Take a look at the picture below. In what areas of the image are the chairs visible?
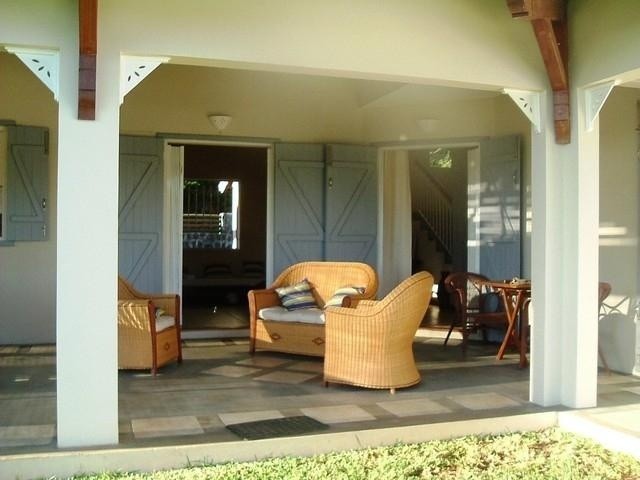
[118,277,182,380]
[442,271,508,355]
[323,270,434,390]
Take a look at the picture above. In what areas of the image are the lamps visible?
[209,115,231,130]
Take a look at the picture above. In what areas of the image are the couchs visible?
[248,260,378,361]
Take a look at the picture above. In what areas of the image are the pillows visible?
[201,261,233,278]
[274,277,364,314]
[243,258,264,278]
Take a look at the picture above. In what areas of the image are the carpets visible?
[226,414,326,439]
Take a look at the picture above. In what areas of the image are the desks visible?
[478,279,532,371]
